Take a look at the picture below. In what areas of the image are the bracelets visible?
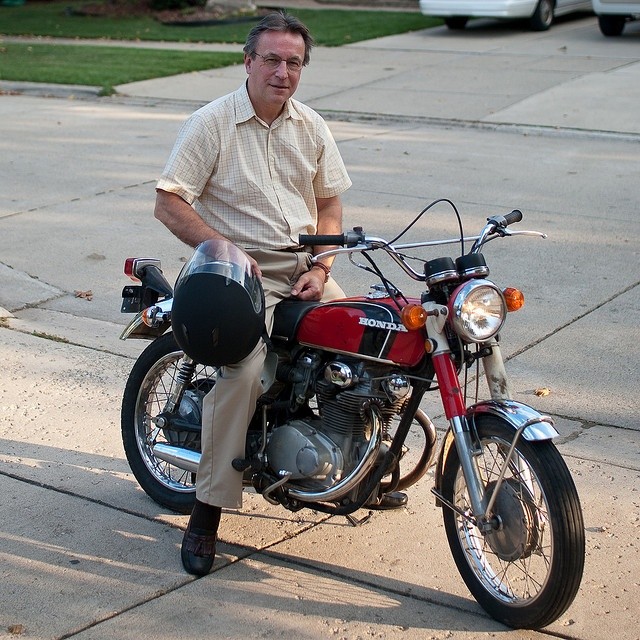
[313,262,329,284]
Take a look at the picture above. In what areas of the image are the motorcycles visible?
[119,199,585,629]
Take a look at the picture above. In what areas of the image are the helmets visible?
[171,239,265,366]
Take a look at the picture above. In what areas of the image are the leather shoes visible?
[181,500,221,575]
[382,491,407,506]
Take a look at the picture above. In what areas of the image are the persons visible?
[155,12,342,576]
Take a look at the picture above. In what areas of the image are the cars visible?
[419,0,595,31]
[593,0,640,35]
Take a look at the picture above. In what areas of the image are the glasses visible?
[251,52,306,73]
